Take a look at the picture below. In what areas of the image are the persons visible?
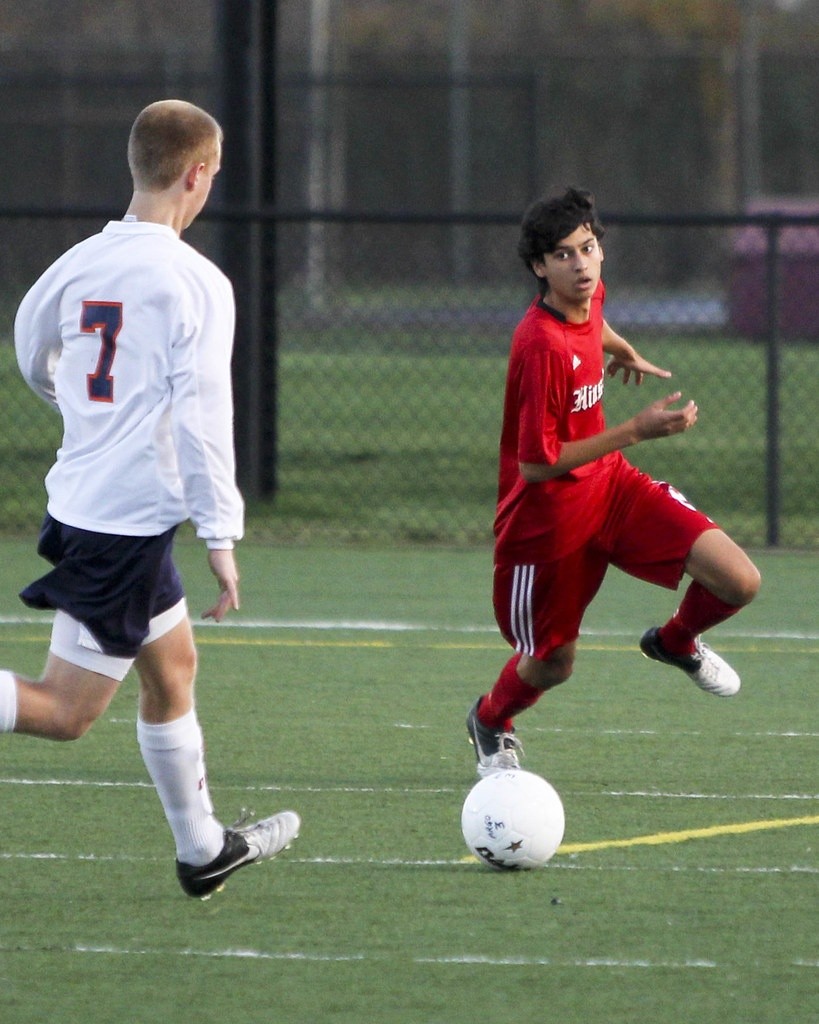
[466,188,763,779]
[0,98,302,899]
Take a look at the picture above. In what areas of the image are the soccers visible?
[461,770,565,869]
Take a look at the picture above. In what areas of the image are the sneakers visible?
[176,807,301,901]
[463,695,525,784]
[638,626,741,697]
